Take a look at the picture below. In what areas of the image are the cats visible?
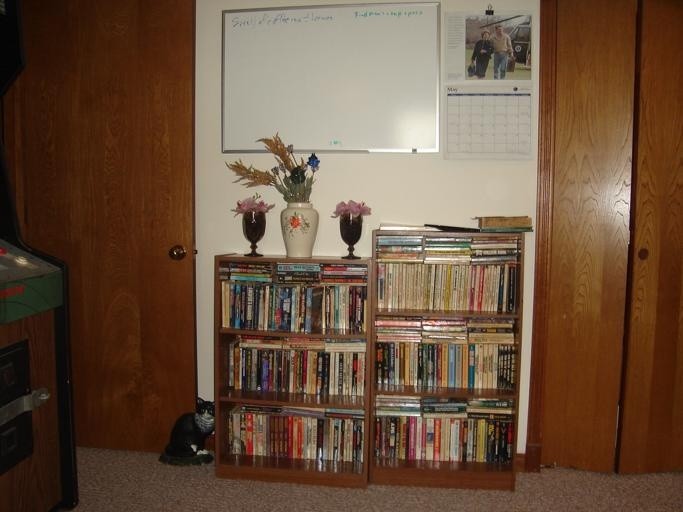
[158,396,215,466]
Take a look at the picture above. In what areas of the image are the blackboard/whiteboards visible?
[220,3,441,155]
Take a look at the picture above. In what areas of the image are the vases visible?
[280,203,319,258]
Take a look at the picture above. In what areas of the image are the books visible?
[373,394,517,465]
[223,403,365,464]
[374,317,518,391]
[223,334,367,407]
[376,215,533,263]
[376,261,520,314]
[219,261,369,334]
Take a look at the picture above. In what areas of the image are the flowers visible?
[222,132,372,218]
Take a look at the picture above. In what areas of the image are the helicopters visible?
[479,14,532,68]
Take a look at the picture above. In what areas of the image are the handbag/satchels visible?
[467,64,476,76]
[508,59,516,73]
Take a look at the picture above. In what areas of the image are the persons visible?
[489,25,515,80]
[467,30,492,78]
